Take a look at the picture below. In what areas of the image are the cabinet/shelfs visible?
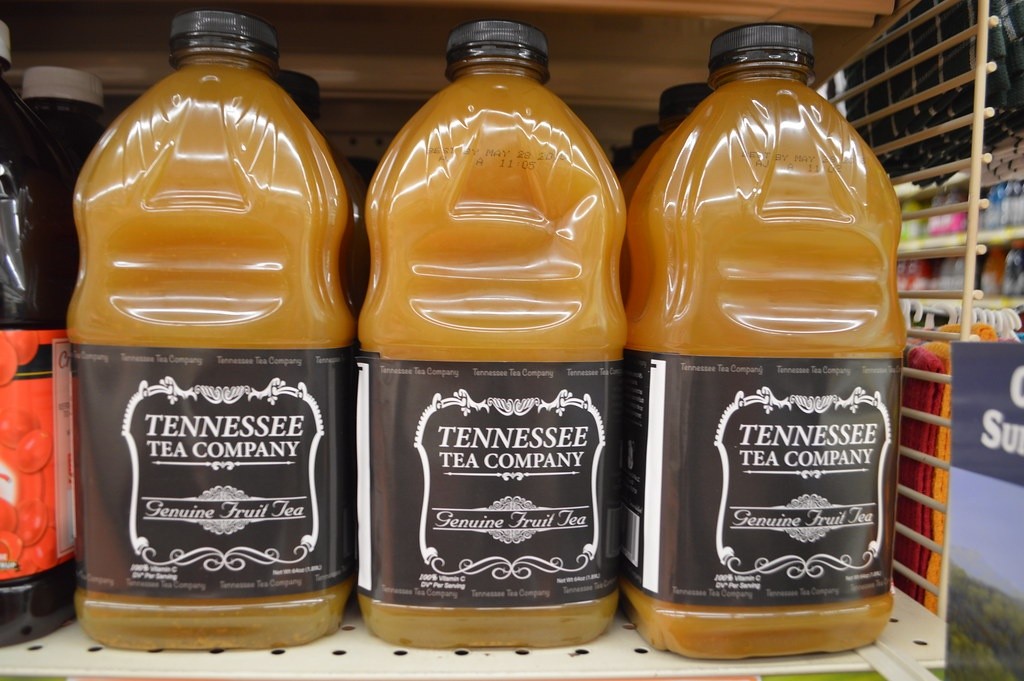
[893,160,1024,310]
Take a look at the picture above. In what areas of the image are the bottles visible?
[0,8,901,659]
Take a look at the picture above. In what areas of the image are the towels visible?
[837,1,1022,182]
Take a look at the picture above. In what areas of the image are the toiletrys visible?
[896,184,1024,294]
